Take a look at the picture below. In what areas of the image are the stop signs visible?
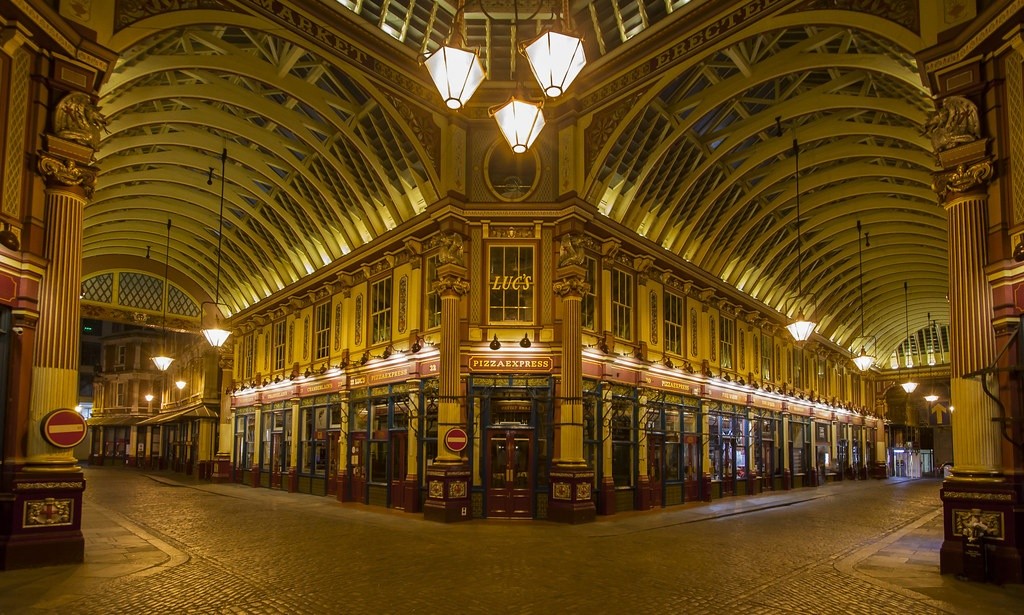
[445,427,468,452]
[43,407,88,449]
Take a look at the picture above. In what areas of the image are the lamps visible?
[490,333,502,350]
[599,130,957,420]
[144,145,429,399]
[417,0,590,156]
[517,331,532,349]
